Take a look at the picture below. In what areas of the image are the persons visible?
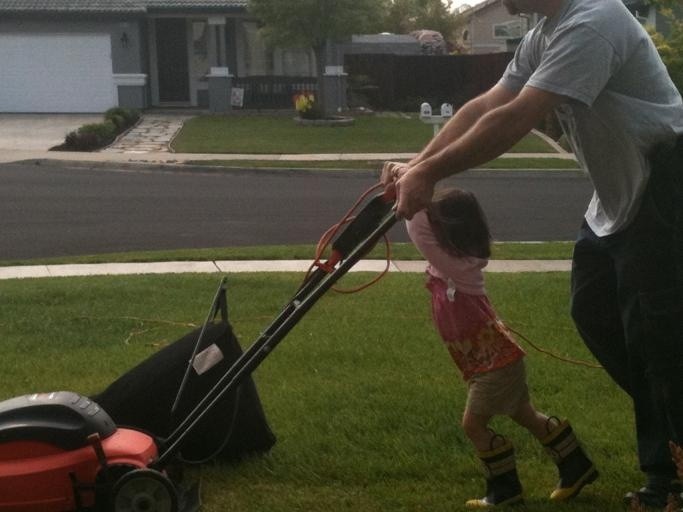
[391,165,598,508]
[382,0,683,512]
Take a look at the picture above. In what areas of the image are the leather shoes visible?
[624,486,682,509]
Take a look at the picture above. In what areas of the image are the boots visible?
[538,416,600,501]
[465,428,524,509]
